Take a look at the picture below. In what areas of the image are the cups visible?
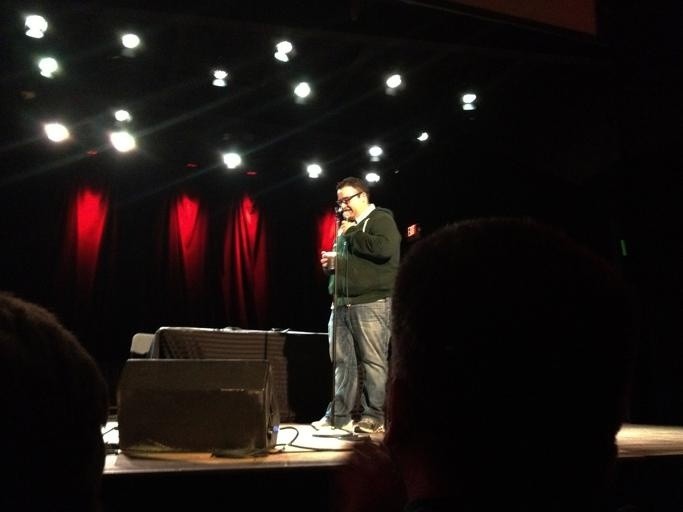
[324,251,338,270]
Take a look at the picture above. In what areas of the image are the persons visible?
[0,293,109,512]
[310,176,401,434]
[342,214,626,510]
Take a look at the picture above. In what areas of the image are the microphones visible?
[342,210,352,223]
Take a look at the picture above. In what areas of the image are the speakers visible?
[115,358,282,455]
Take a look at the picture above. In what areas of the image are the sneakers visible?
[311,416,354,431]
[354,416,385,434]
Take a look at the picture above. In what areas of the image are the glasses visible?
[335,192,362,205]
